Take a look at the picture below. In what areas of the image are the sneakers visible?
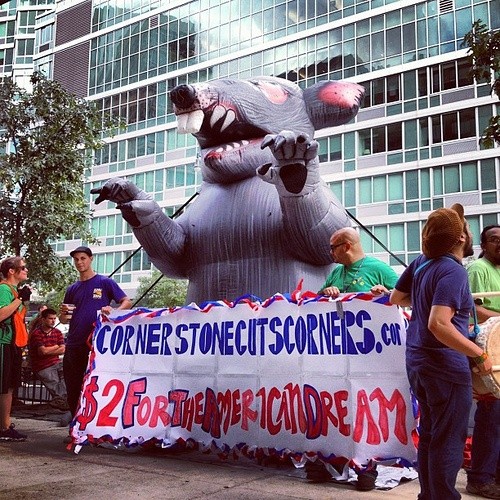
[0,428,28,441]
[306,463,346,483]
[356,469,379,490]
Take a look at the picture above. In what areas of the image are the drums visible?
[468,315,500,401]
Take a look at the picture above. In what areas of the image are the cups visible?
[61,304,74,320]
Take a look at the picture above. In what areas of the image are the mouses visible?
[90,75,365,309]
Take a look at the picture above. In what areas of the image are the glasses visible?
[330,243,347,251]
[14,263,27,271]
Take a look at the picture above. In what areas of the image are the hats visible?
[70,246,92,258]
[422,203,464,258]
